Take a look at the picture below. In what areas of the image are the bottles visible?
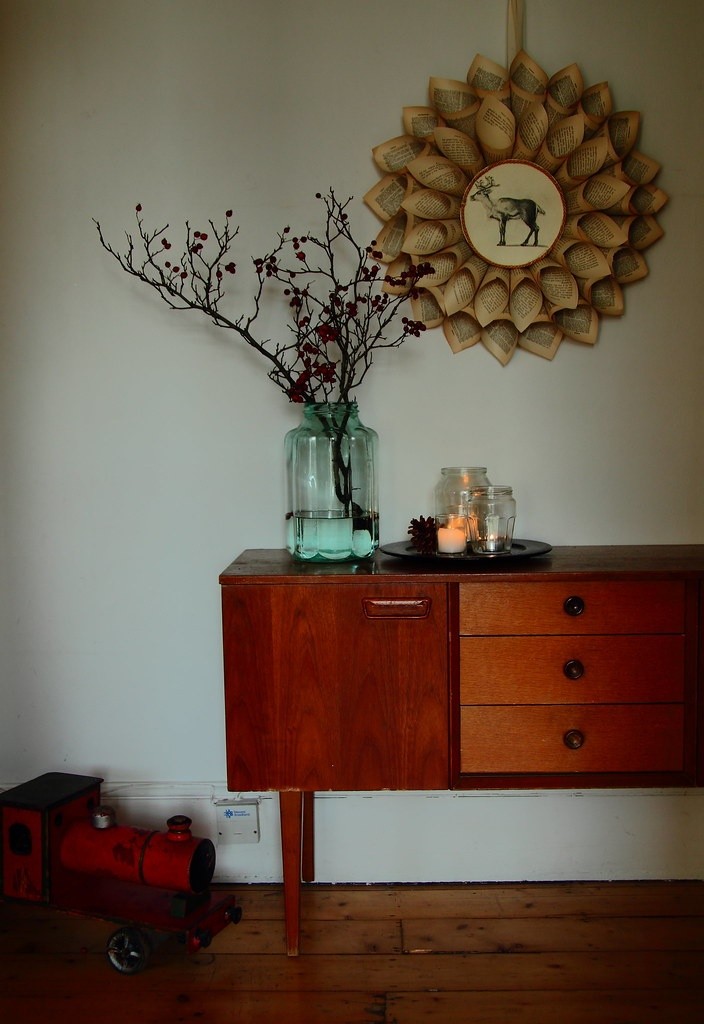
[467,486,517,555]
[434,465,493,545]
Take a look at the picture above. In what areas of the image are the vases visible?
[278,400,386,568]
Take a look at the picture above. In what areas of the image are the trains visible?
[0,772,242,974]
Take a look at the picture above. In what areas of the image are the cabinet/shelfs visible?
[216,543,703,960]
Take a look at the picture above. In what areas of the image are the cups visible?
[435,512,468,557]
